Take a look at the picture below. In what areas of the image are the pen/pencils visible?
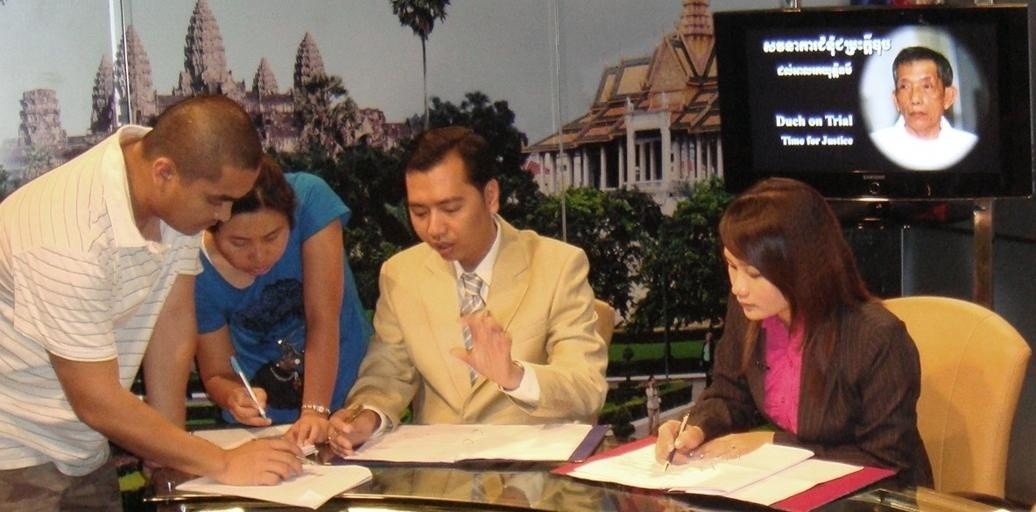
[229,355,268,421]
[328,402,364,441]
[664,409,692,472]
[295,456,320,467]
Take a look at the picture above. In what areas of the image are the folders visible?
[550,435,896,512]
[323,423,611,465]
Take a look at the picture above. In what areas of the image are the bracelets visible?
[298,402,331,415]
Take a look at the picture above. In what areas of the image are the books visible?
[174,458,375,512]
[345,419,594,462]
[191,428,326,460]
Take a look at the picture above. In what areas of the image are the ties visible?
[459,274,486,386]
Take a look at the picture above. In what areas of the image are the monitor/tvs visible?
[713,3,1036,202]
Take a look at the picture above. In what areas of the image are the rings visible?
[730,441,738,449]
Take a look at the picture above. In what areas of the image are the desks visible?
[0,425,1000,512]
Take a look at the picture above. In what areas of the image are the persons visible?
[652,174,938,492]
[324,122,611,463]
[0,89,312,512]
[190,153,371,452]
[865,39,983,175]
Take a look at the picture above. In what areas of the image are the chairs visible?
[867,293,1036,512]
